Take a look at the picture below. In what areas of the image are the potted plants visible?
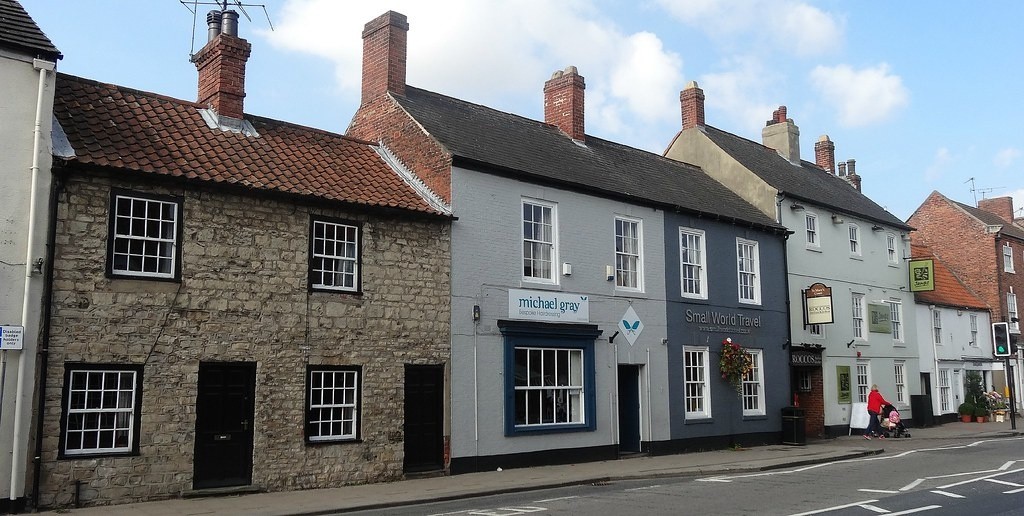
[975,408,985,423]
[982,410,990,423]
[958,402,974,423]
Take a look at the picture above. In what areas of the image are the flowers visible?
[713,338,754,397]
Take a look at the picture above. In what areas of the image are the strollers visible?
[877,404,912,438]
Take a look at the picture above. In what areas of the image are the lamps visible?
[788,201,811,211]
[803,343,810,348]
[832,213,844,225]
[995,237,1003,242]
[871,225,885,231]
[901,232,914,242]
[1003,317,1021,325]
[812,344,823,348]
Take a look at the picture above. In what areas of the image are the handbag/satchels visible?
[889,410,900,423]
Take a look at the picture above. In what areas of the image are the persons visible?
[981,391,1000,405]
[863,384,893,441]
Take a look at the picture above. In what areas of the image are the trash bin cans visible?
[781,406,807,446]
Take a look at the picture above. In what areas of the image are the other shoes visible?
[863,434,871,440]
[879,435,885,440]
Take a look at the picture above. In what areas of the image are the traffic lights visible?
[1009,333,1019,355]
[992,322,1012,357]
